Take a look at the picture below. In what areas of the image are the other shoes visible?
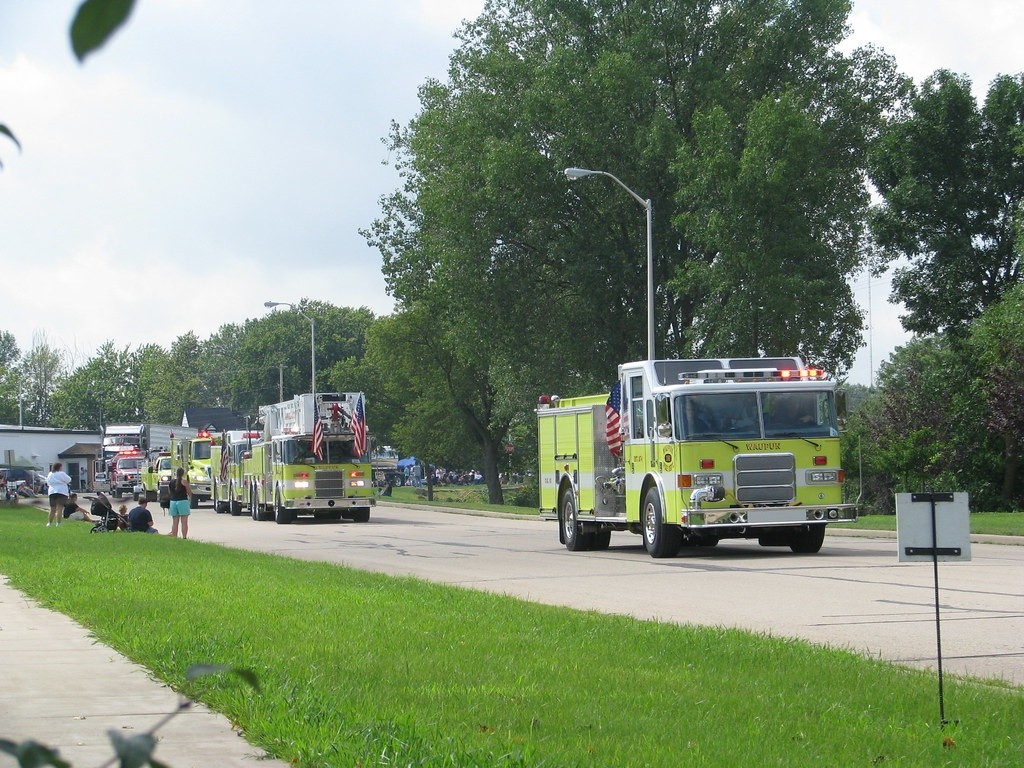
[46,524,51,527]
[56,525,60,527]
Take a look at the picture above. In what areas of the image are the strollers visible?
[83,491,132,533]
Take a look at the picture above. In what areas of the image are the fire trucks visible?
[244,388,371,524]
[533,356,863,558]
[94,393,263,516]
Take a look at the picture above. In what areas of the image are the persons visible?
[404,461,483,488]
[80,467,88,493]
[498,472,519,487]
[168,467,192,539]
[129,498,158,534]
[0,476,40,505]
[63,493,100,522]
[46,462,72,527]
[118,505,129,531]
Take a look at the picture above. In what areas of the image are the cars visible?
[376,468,406,487]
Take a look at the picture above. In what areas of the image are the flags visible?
[349,391,368,460]
[311,390,323,462]
[219,431,228,483]
[605,379,624,458]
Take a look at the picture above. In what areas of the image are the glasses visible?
[75,497,77,498]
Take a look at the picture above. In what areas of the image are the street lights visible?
[264,301,316,394]
[565,168,655,360]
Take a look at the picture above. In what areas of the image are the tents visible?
[397,456,435,479]
